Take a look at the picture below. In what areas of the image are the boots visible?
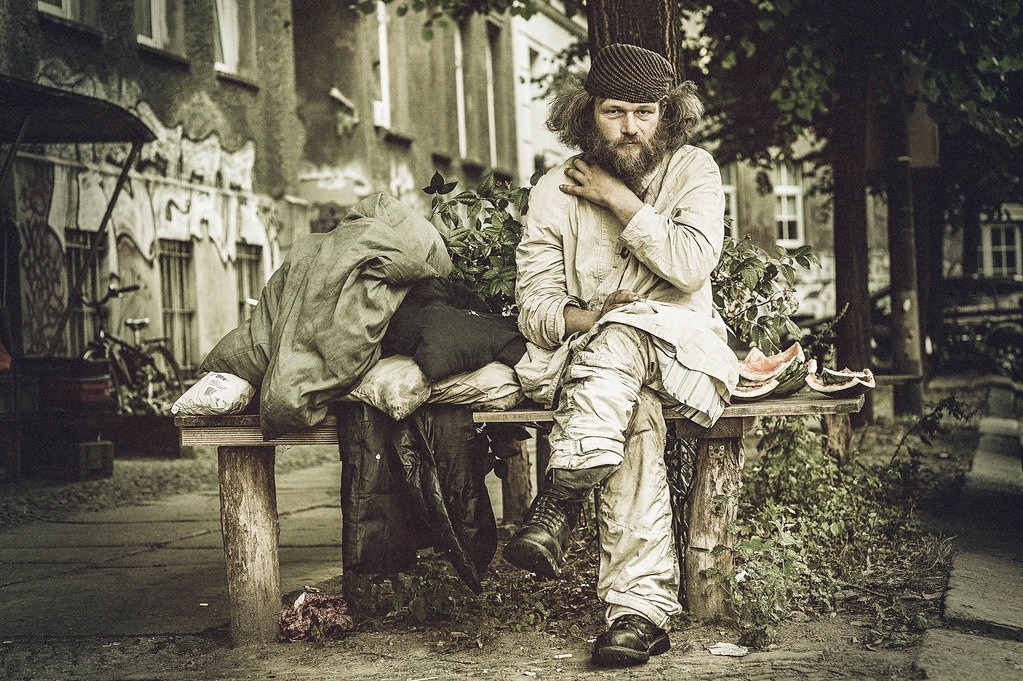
[501,468,588,579]
[591,614,671,666]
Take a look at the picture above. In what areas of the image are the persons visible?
[504,43,740,663]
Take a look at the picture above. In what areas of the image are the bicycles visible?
[76,282,187,418]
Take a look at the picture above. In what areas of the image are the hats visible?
[584,43,674,104]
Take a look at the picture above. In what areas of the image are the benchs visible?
[175,388,867,649]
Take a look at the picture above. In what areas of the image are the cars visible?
[775,270,1023,382]
